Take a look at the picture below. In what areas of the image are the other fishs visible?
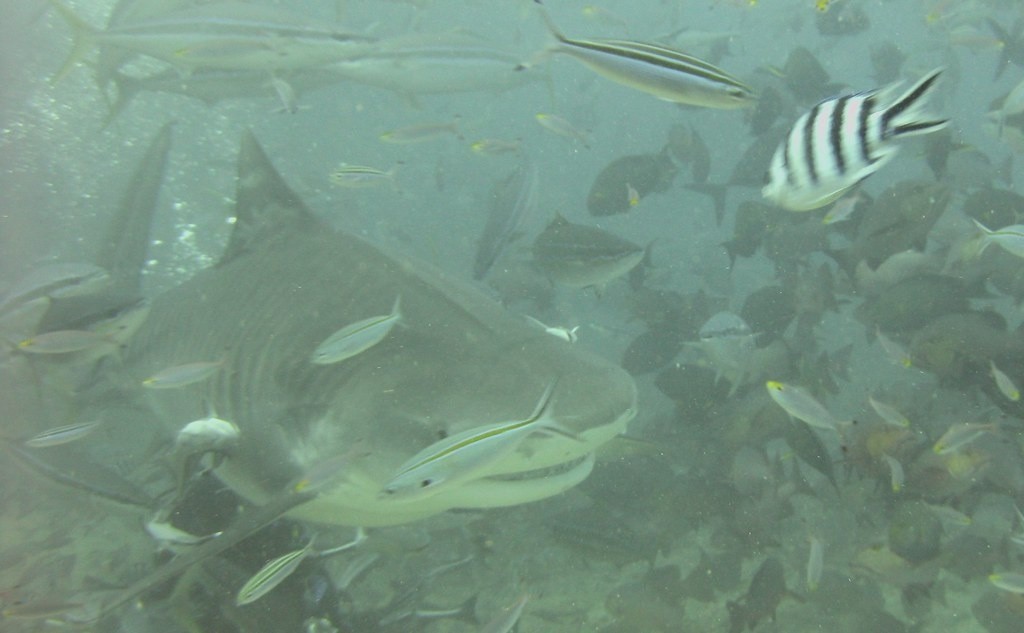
[0,0,1024,633]
